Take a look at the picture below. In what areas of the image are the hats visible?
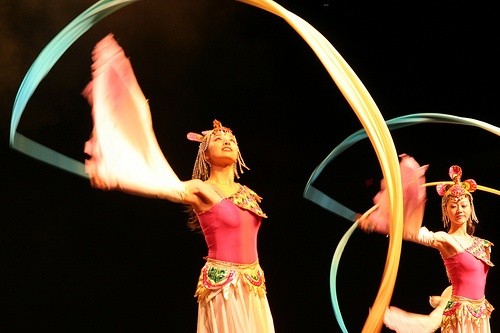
[436,164,479,223]
[186,118,250,179]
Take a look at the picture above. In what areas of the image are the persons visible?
[361,154,495,333]
[84,32,276,333]
